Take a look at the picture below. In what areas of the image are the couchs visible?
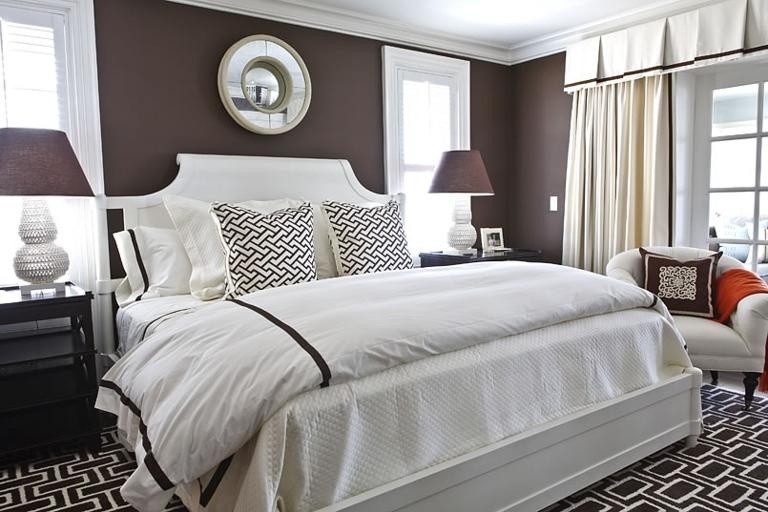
[605,244,768,409]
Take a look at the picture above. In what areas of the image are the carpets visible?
[2,379,768,511]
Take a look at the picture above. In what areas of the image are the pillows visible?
[321,200,409,277]
[208,199,317,302]
[162,192,335,301]
[115,225,189,307]
[637,246,724,315]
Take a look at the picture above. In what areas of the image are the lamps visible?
[428,149,496,255]
[0,127,95,299]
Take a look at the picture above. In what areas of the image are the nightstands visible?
[419,250,540,269]
[0,282,100,467]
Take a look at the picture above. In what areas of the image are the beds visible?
[95,155,703,511]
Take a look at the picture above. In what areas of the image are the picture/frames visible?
[478,227,504,252]
